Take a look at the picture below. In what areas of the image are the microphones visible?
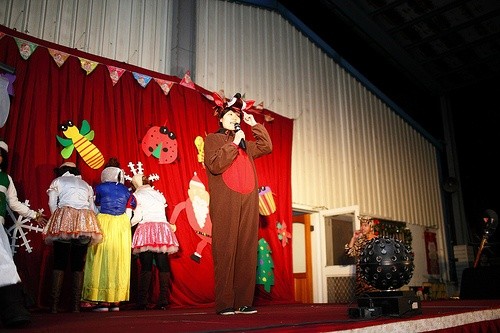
[234,123,246,149]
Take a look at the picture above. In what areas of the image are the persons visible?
[344,213,378,294]
[83,158,136,313]
[42,162,102,316]
[130,172,179,310]
[0,140,48,326]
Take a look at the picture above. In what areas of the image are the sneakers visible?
[234,306,257,314]
[220,309,235,315]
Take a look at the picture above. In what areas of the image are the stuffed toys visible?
[204,102,274,315]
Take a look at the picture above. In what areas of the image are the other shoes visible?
[110,306,120,311]
[0,284,31,326]
[93,308,109,311]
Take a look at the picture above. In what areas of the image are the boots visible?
[72,272,84,312]
[136,271,151,310]
[155,271,170,309]
[50,270,64,313]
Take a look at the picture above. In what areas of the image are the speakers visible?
[460,264,500,300]
